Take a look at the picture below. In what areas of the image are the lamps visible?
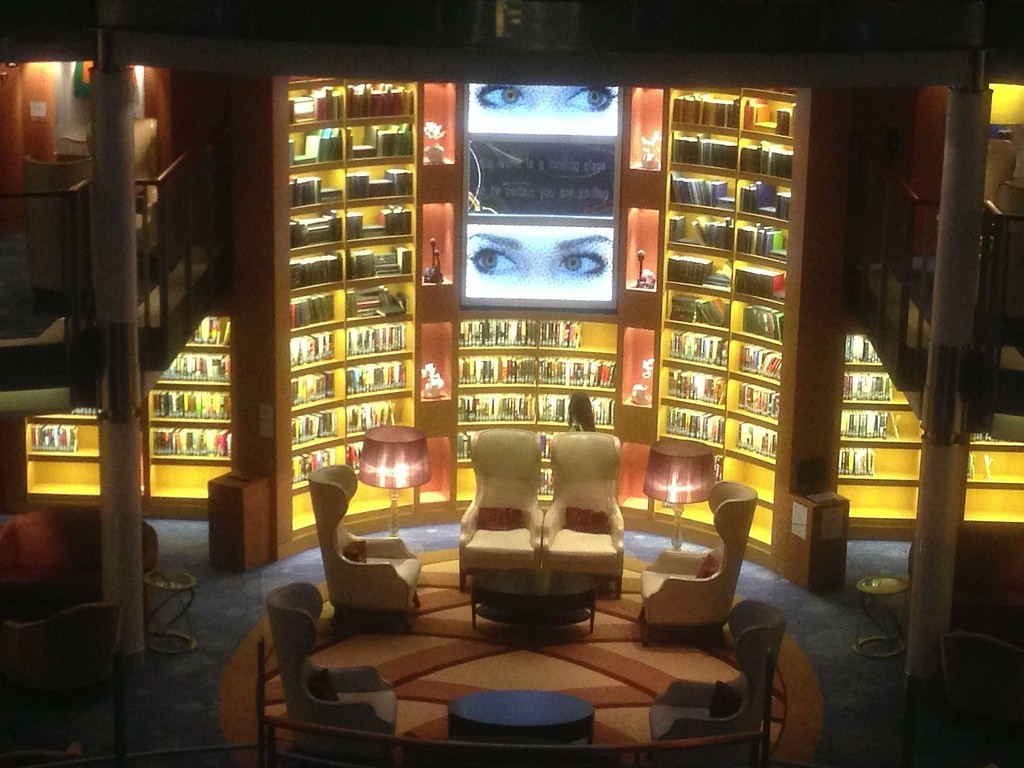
[357,426,430,537]
[643,439,716,554]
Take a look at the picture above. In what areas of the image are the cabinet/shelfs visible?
[230,74,850,565]
[838,334,1024,538]
[25,316,231,497]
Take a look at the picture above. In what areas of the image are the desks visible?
[852,575,908,658]
[469,566,598,633]
[446,688,595,744]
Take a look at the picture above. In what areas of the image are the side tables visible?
[142,567,199,653]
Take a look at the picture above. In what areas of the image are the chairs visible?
[457,428,625,599]
[905,524,1024,622]
[648,598,789,768]
[940,633,1024,740]
[267,582,397,764]
[639,481,758,649]
[1,602,121,760]
[308,465,423,635]
[0,502,158,616]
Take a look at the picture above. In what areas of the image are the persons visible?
[468,83,619,137]
[465,224,615,301]
[569,392,595,433]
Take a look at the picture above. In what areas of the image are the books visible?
[141,315,231,456]
[287,76,412,483]
[460,321,614,499]
[838,335,893,475]
[31,424,79,451]
[967,432,999,482]
[666,90,797,463]
